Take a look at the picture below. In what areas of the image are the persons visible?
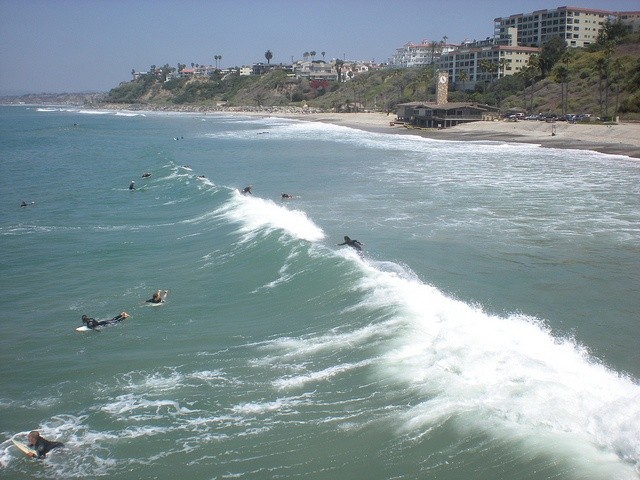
[24,431,65,460]
[21,201,28,206]
[140,172,151,177]
[241,184,254,196]
[80,312,129,329]
[335,235,364,253]
[280,192,294,200]
[128,180,135,190]
[146,289,169,304]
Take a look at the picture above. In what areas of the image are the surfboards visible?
[76,321,115,331]
[144,302,164,308]
[11,438,38,460]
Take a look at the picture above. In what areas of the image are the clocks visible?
[440,75,447,84]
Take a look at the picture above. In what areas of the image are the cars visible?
[504,112,601,122]
